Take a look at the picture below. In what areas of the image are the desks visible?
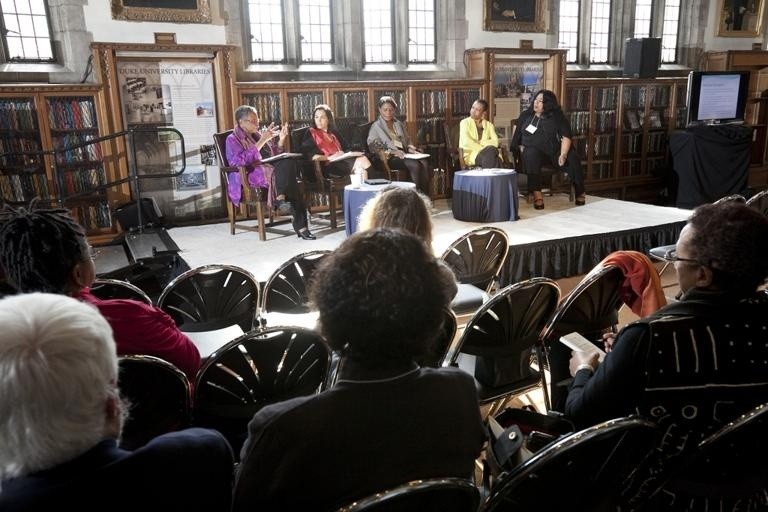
[668,123,755,209]
[452,167,521,223]
[343,180,416,235]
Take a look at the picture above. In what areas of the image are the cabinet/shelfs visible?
[232,78,491,220]
[0,82,133,247]
[565,77,692,193]
[704,50,768,172]
[464,48,570,192]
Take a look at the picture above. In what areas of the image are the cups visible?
[350,174,362,189]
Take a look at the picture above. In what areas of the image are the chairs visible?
[510,119,574,204]
[443,118,509,170]
[212,128,306,240]
[286,125,351,229]
[358,121,434,208]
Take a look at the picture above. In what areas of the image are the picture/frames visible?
[714,0,767,38]
[483,0,546,33]
[110,0,212,25]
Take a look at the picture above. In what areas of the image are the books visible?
[623,86,681,176]
[0,98,112,231]
[244,93,322,144]
[334,90,407,119]
[416,89,480,195]
[556,328,607,364]
[248,184,343,214]
[564,87,619,180]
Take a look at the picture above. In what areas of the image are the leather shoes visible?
[575,196,585,205]
[534,199,545,209]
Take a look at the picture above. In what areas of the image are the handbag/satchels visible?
[483,403,576,491]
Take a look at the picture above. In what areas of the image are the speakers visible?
[624,38,662,79]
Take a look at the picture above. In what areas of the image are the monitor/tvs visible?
[686,69,751,128]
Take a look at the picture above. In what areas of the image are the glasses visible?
[664,248,699,264]
[79,244,102,263]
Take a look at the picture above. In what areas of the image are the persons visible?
[356,185,435,249]
[222,103,319,241]
[459,99,504,168]
[0,291,235,511]
[299,103,380,186]
[367,95,430,197]
[0,192,201,378]
[567,201,767,444]
[510,90,586,210]
[234,227,487,512]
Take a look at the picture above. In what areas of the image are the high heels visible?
[296,229,316,240]
[272,199,292,214]
